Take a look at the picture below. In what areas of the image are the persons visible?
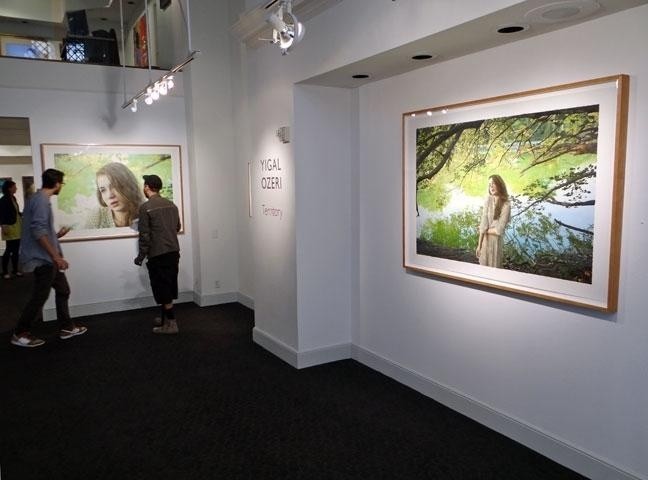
[476,175,510,268]
[134,174,181,334]
[9,169,88,348]
[0,181,23,281]
[85,162,146,230]
[134,27,142,66]
[24,180,35,198]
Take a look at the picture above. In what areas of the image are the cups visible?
[59,258,68,273]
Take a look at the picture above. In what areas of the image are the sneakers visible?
[11,332,46,347]
[153,319,179,334]
[60,326,87,340]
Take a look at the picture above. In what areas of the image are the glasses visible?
[59,182,66,187]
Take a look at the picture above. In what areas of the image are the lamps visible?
[265,0,305,57]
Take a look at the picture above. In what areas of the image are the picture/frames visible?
[400,72,629,317]
[39,142,185,244]
[130,6,151,67]
